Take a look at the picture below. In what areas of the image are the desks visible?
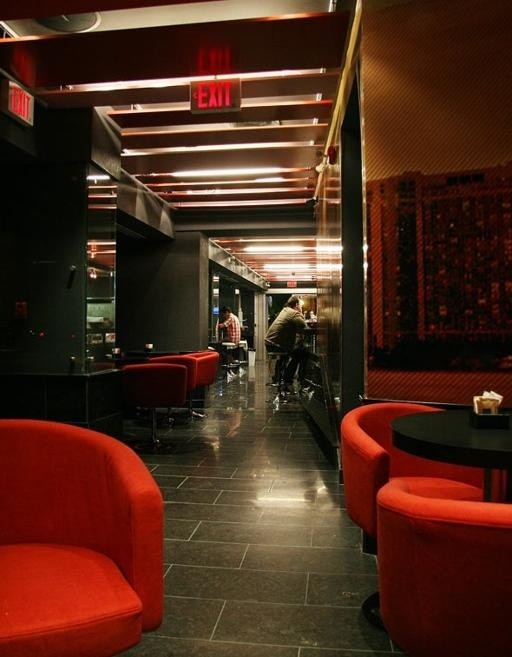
[391,407,510,503]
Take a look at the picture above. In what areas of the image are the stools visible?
[221,341,248,381]
[267,351,290,405]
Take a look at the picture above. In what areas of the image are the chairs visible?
[118,349,221,440]
[340,401,503,557]
[375,476,511,656]
[0,419,165,657]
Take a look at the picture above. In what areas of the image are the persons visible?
[218,305,241,363]
[265,295,307,383]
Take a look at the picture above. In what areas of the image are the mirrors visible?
[212,269,220,347]
[86,152,117,372]
